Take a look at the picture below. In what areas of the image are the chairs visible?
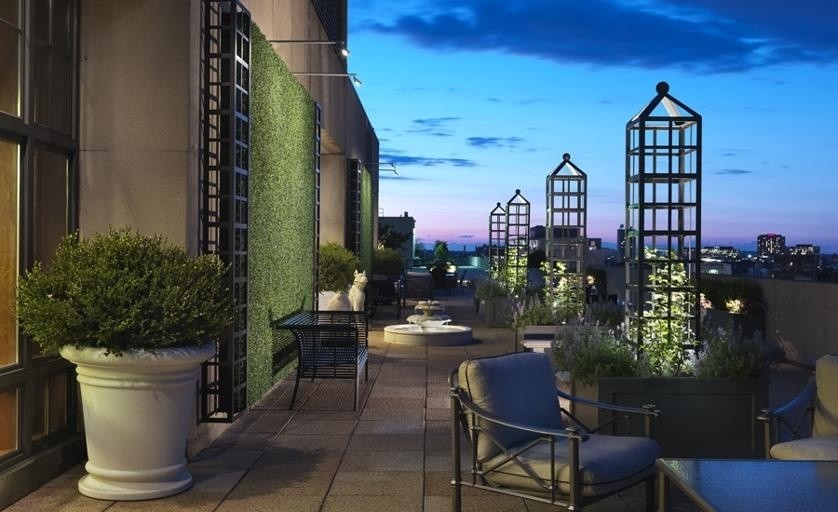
[280,304,370,412]
[441,349,664,512]
[368,260,468,320]
[756,352,838,461]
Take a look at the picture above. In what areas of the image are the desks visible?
[654,455,838,512]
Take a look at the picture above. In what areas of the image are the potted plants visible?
[314,241,357,310]
[17,225,238,498]
